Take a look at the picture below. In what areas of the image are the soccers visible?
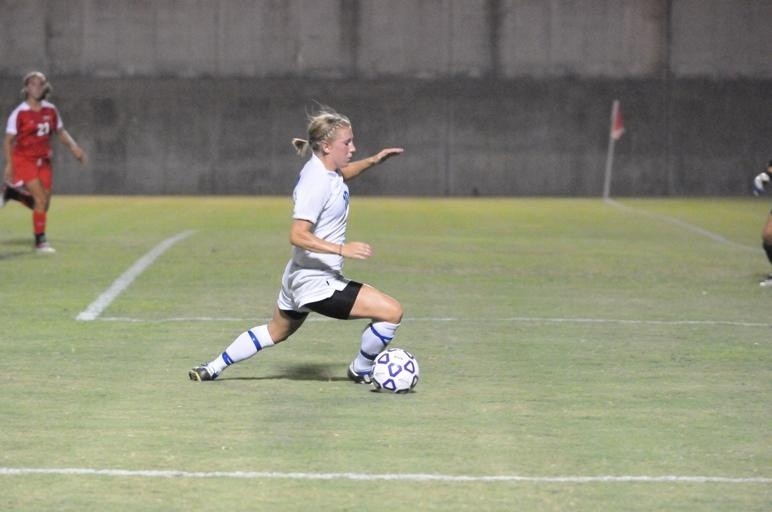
[370,347,419,394]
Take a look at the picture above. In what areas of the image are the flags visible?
[610,102,626,141]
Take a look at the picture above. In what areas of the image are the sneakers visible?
[760,273,772,288]
[189,366,215,382]
[346,363,373,385]
[0,182,10,208]
[33,243,57,254]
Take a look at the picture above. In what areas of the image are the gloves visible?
[751,173,771,197]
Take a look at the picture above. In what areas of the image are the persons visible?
[0,71,88,254]
[188,94,408,387]
[748,157,771,289]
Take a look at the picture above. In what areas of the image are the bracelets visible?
[339,244,343,256]
